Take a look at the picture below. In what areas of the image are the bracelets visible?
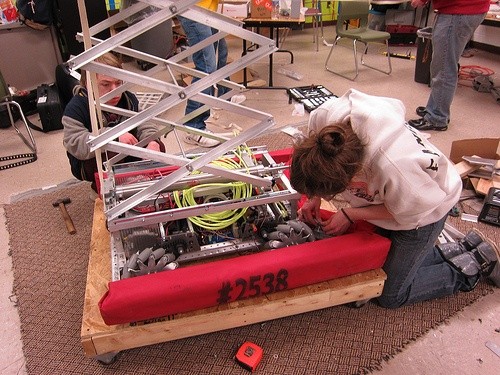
[342,208,354,224]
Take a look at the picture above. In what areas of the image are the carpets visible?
[4,125,500,375]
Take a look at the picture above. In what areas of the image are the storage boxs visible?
[251,0,279,47]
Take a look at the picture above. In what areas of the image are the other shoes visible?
[473,238,500,289]
[463,227,486,249]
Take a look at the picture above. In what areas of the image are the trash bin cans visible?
[121,0,175,70]
[415,26,432,84]
[368,10,385,31]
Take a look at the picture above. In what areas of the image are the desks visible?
[305,13,323,52]
[240,17,306,90]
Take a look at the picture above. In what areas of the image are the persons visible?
[177,0,246,147]
[62,53,166,203]
[407,0,492,132]
[291,87,500,309]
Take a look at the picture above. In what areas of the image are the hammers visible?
[52,197,76,234]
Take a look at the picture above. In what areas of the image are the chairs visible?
[0,71,37,154]
[325,0,391,81]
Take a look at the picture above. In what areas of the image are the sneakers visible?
[415,106,450,124]
[211,94,247,110]
[182,128,222,148]
[408,116,449,132]
